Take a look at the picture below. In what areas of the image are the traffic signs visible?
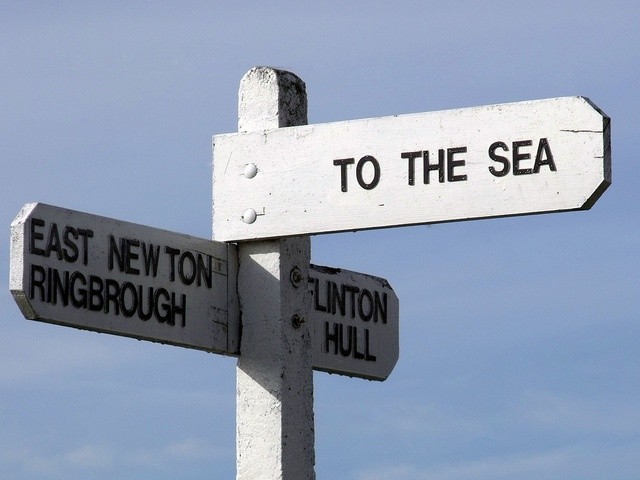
[212,96,612,243]
[9,201,398,381]
